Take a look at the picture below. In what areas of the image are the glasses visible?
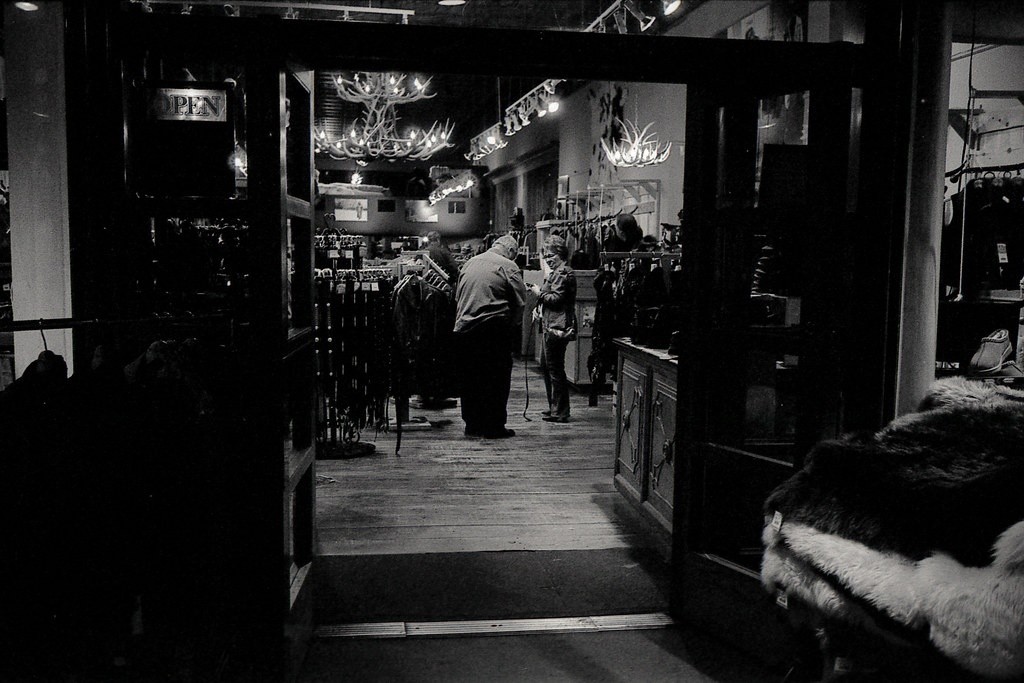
[543,252,558,260]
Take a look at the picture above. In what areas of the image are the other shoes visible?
[485,427,515,439]
[463,423,485,437]
[541,410,552,416]
[542,417,568,423]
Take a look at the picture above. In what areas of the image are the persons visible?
[531,236,577,424]
[612,213,649,253]
[453,236,527,440]
[423,231,456,283]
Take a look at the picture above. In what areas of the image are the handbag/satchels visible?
[544,291,578,343]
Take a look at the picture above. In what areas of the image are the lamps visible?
[661,0,681,16]
[542,80,561,94]
[463,133,508,160]
[510,112,522,131]
[530,98,547,118]
[538,93,559,113]
[599,83,672,168]
[314,0,456,161]
[625,0,655,32]
[504,117,516,137]
[518,107,530,126]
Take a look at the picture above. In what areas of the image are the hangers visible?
[394,264,430,294]
[38,318,54,360]
[423,259,451,300]
[144,311,202,377]
[603,250,683,272]
